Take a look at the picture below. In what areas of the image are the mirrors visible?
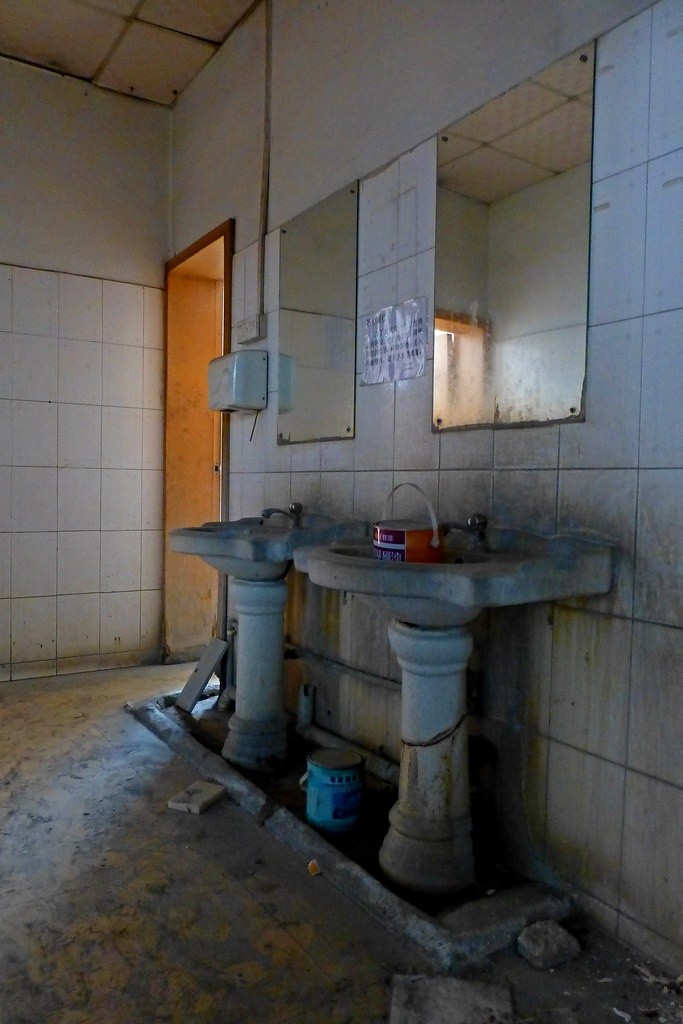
[431,39,596,432]
[276,179,360,446]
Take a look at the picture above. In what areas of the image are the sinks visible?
[166,517,366,581]
[290,537,614,623]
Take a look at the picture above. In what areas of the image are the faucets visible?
[458,513,486,548]
[260,502,301,527]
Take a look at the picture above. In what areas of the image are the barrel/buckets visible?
[372,482,444,564]
[299,748,364,834]
